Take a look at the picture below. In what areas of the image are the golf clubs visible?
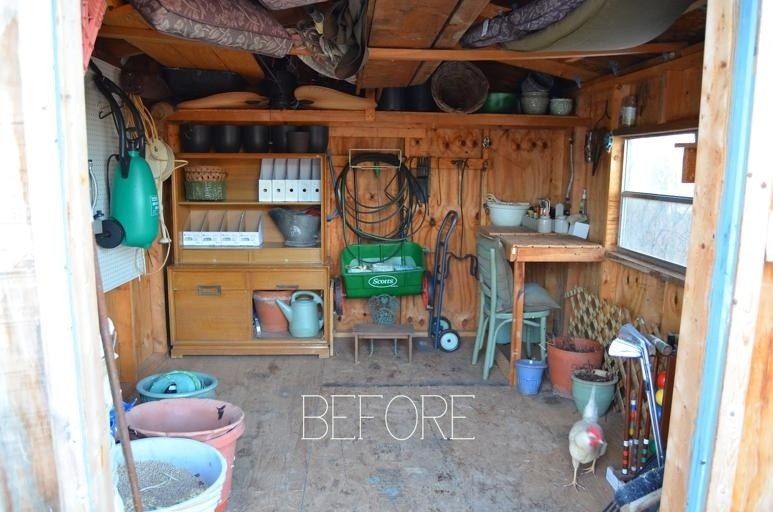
[608,322,663,468]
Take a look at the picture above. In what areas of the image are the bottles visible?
[621,96,636,128]
[528,197,550,219]
[563,192,570,215]
[536,214,552,234]
[578,188,587,216]
[554,216,568,233]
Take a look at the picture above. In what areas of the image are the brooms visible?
[612,467,665,512]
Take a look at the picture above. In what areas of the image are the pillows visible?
[503,281,562,312]
[475,233,514,305]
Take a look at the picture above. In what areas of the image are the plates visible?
[151,371,202,393]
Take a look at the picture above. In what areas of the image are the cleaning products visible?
[578,189,586,214]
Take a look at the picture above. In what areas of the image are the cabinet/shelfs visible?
[166,154,327,359]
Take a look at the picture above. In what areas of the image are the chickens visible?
[564,384,608,494]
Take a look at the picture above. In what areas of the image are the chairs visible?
[472,243,550,380]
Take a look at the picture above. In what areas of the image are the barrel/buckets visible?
[123,397,246,512]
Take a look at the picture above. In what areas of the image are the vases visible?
[253,290,294,332]
[180,123,329,154]
[431,59,573,115]
[547,337,603,394]
[111,370,247,512]
[571,369,618,417]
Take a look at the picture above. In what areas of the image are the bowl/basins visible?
[486,200,529,226]
[122,397,245,441]
[112,437,226,512]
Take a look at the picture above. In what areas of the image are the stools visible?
[352,324,415,362]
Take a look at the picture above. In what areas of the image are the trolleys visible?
[410,210,480,354]
[88,60,161,250]
[332,147,433,319]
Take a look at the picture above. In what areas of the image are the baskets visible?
[431,60,489,114]
[184,165,227,200]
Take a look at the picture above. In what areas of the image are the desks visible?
[475,225,607,386]
